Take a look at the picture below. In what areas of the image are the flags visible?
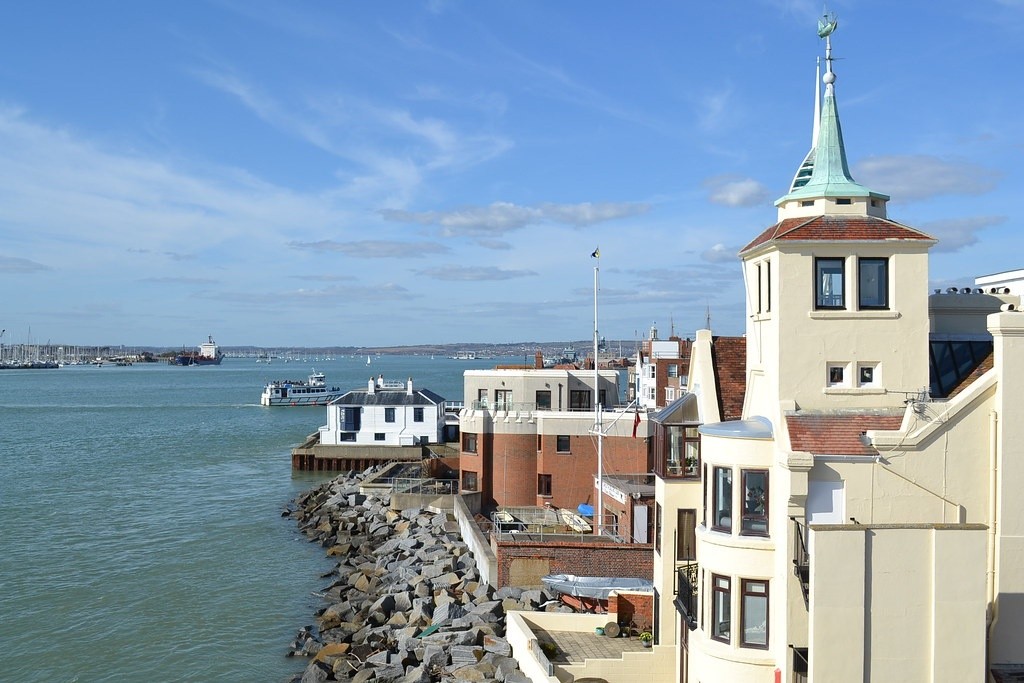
[591,249,599,258]
[632,412,641,439]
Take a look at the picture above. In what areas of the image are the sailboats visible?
[375,351,380,360]
[265,353,272,362]
[0,326,59,369]
[61,345,104,365]
[341,352,363,359]
[431,355,434,359]
[256,353,261,362]
[279,347,336,363]
[414,352,418,355]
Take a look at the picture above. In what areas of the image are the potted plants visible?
[745,487,762,513]
[680,457,693,472]
[638,631,652,646]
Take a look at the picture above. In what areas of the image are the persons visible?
[282,380,304,386]
[332,387,340,391]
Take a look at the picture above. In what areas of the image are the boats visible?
[541,574,653,600]
[366,355,371,367]
[117,359,132,366]
[490,511,514,523]
[175,349,198,365]
[561,508,592,533]
[195,335,225,365]
[261,370,344,406]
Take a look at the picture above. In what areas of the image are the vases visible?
[596,627,604,635]
